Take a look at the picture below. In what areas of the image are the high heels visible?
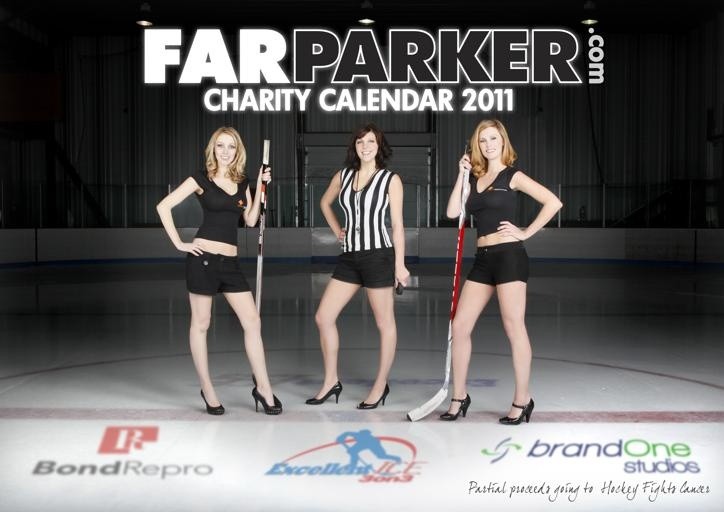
[305,380,343,405]
[251,386,282,416]
[356,383,390,410]
[497,397,535,426]
[439,393,471,421]
[200,388,225,416]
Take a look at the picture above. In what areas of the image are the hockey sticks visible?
[406,139,470,422]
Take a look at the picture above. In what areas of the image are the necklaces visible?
[355,168,380,233]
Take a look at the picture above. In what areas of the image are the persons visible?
[440,119,564,425]
[156,126,283,415]
[305,121,411,410]
